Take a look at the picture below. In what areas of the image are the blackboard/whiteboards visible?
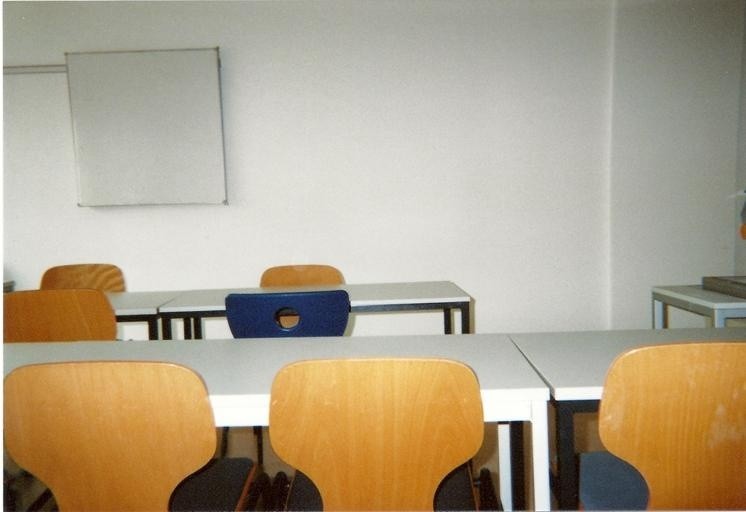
[64,46,227,207]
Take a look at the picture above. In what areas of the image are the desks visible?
[509,328,746,504]
[648,281,746,330]
[159,280,477,337]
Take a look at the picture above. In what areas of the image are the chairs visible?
[224,263,355,340]
[4,263,127,340]
[3,339,746,509]
